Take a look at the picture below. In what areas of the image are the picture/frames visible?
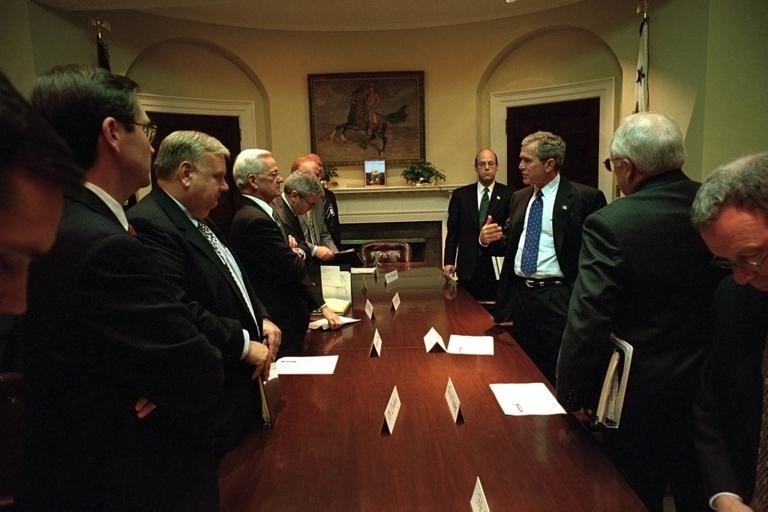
[305,69,427,169]
[363,160,388,187]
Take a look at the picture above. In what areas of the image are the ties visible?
[198,221,228,267]
[272,210,288,245]
[479,188,489,230]
[521,189,544,277]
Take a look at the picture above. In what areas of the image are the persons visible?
[362,78,379,140]
[442,148,519,301]
[267,168,327,291]
[0,70,84,319]
[26,64,233,510]
[227,148,343,343]
[292,157,341,264]
[126,129,286,467]
[304,153,342,255]
[687,149,767,511]
[477,130,608,394]
[552,113,717,510]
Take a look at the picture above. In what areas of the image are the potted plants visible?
[399,158,447,186]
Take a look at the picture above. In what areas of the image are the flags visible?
[632,18,649,113]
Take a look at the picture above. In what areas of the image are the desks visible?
[215,256,650,511]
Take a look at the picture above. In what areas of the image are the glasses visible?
[710,254,768,271]
[131,122,158,143]
[602,158,623,171]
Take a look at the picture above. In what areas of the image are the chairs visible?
[358,239,413,272]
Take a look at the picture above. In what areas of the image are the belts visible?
[519,279,562,288]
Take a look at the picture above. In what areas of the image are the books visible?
[309,262,354,319]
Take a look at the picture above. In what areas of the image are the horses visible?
[326,87,410,157]
